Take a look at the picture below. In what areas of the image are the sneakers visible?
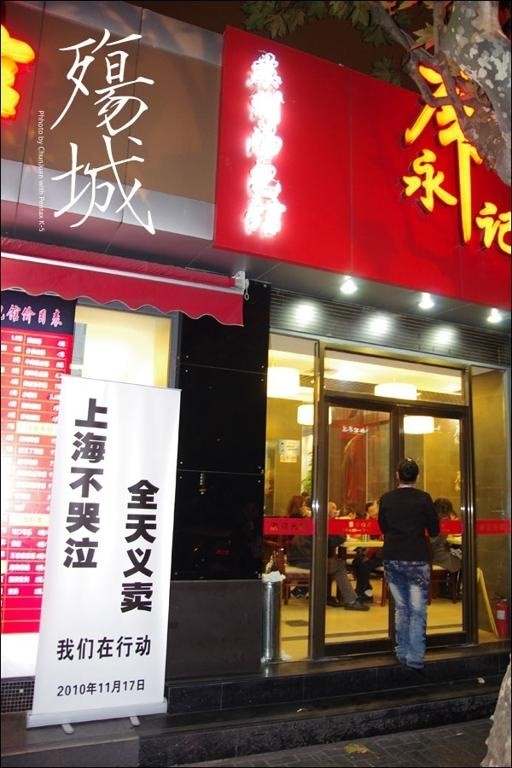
[397,656,424,669]
[288,585,374,611]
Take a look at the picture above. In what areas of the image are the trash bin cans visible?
[260,580,281,662]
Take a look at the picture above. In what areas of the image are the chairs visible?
[427,535,457,605]
[276,535,310,605]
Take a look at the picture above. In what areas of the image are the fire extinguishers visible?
[490,593,509,639]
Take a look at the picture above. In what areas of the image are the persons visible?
[281,489,464,610]
[378,456,442,675]
